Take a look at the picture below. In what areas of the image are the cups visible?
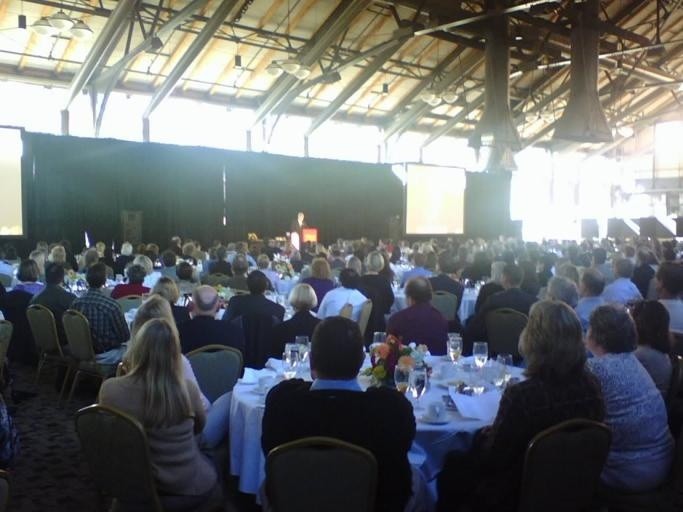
[372,332,386,344]
[282,352,298,379]
[440,363,459,380]
[429,401,445,419]
[258,376,273,391]
[284,343,298,362]
[410,367,427,412]
[473,342,487,370]
[446,333,460,361]
[450,337,462,366]
[394,365,410,396]
[497,354,512,379]
[296,336,309,362]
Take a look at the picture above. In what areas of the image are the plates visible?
[251,389,267,396]
[420,415,451,425]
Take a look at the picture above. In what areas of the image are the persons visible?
[249,254,279,291]
[213,240,226,259]
[111,265,150,299]
[387,275,449,356]
[98,318,228,497]
[160,250,176,278]
[623,246,638,265]
[557,263,578,284]
[182,243,197,266]
[301,242,313,263]
[95,240,105,257]
[145,250,160,270]
[222,255,248,291]
[127,294,212,415]
[360,237,368,256]
[427,250,463,322]
[167,236,182,256]
[376,239,393,258]
[148,277,189,323]
[535,256,554,277]
[590,248,611,276]
[78,249,99,274]
[348,256,362,277]
[288,211,309,237]
[147,243,158,253]
[202,248,218,271]
[287,257,302,297]
[662,241,677,261]
[0,244,19,287]
[208,247,230,275]
[116,242,135,264]
[631,250,655,299]
[133,254,161,288]
[481,298,604,512]
[177,285,247,379]
[50,246,66,263]
[361,251,394,352]
[626,298,674,399]
[172,262,192,293]
[408,244,425,266]
[653,261,683,332]
[400,254,432,287]
[228,242,255,267]
[345,241,366,267]
[13,258,45,296]
[538,275,578,311]
[318,268,368,322]
[584,302,675,495]
[67,264,126,365]
[271,284,321,358]
[302,259,337,311]
[30,263,78,343]
[602,257,643,309]
[221,269,285,338]
[574,269,606,329]
[474,261,506,315]
[479,265,535,322]
[261,239,282,258]
[29,250,46,274]
[260,316,421,512]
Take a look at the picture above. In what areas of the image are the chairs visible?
[0,320,13,385]
[185,344,244,402]
[431,290,457,321]
[72,403,162,512]
[61,308,95,405]
[26,303,71,397]
[265,436,379,511]
[359,300,373,338]
[519,418,612,511]
